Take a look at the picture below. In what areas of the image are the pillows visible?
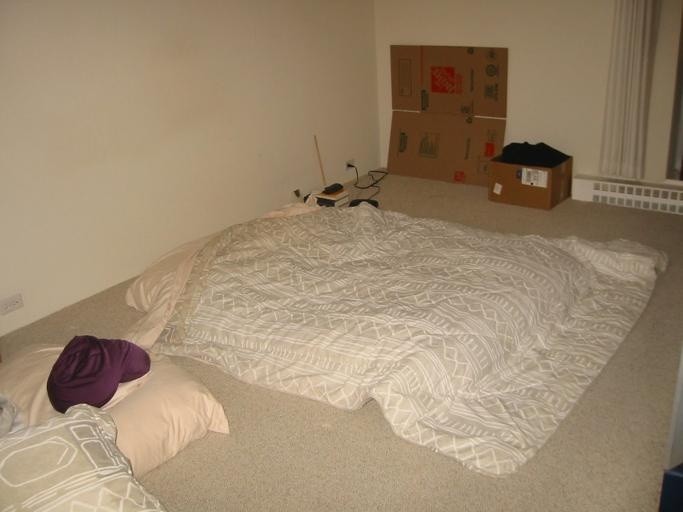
[12,340,230,482]
[1,400,162,510]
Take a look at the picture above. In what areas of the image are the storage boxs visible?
[487,144,573,211]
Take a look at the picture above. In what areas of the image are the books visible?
[316,189,349,207]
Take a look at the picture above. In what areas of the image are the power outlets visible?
[345,158,355,171]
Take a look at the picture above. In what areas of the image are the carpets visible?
[2,169,683,512]
